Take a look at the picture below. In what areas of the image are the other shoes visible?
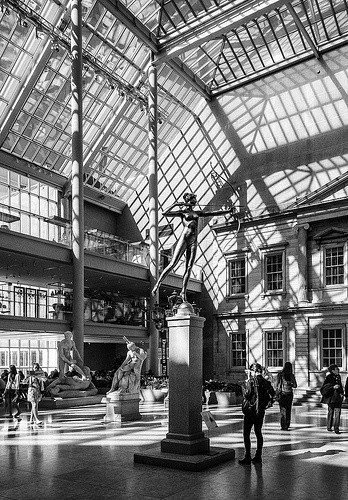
[28,421,34,425]
[37,421,42,425]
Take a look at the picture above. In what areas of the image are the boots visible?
[238,453,251,466]
[251,450,262,463]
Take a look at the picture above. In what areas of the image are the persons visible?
[276,362,297,431]
[238,364,276,464]
[46,331,96,393]
[151,193,236,303]
[320,365,344,434]
[23,363,47,425]
[0,369,154,391]
[106,342,147,395]
[4,364,22,418]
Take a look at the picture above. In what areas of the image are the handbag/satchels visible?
[274,388,282,403]
[242,397,258,416]
